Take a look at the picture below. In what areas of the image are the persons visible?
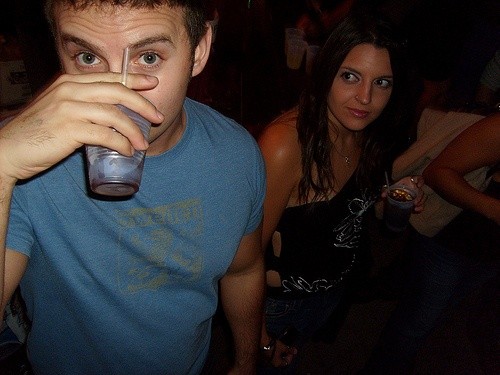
[257,20,432,375]
[357,111,500,375]
[0,0,267,375]
[471,50,500,111]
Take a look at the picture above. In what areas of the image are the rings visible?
[282,357,289,369]
[285,346,290,354]
[405,175,419,186]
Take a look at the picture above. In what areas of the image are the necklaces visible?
[327,135,362,166]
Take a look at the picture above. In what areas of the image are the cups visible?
[306,45,320,76]
[285,28,304,56]
[385,184,419,232]
[286,39,307,70]
[85,104,151,196]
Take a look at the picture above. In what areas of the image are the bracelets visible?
[259,335,277,363]
